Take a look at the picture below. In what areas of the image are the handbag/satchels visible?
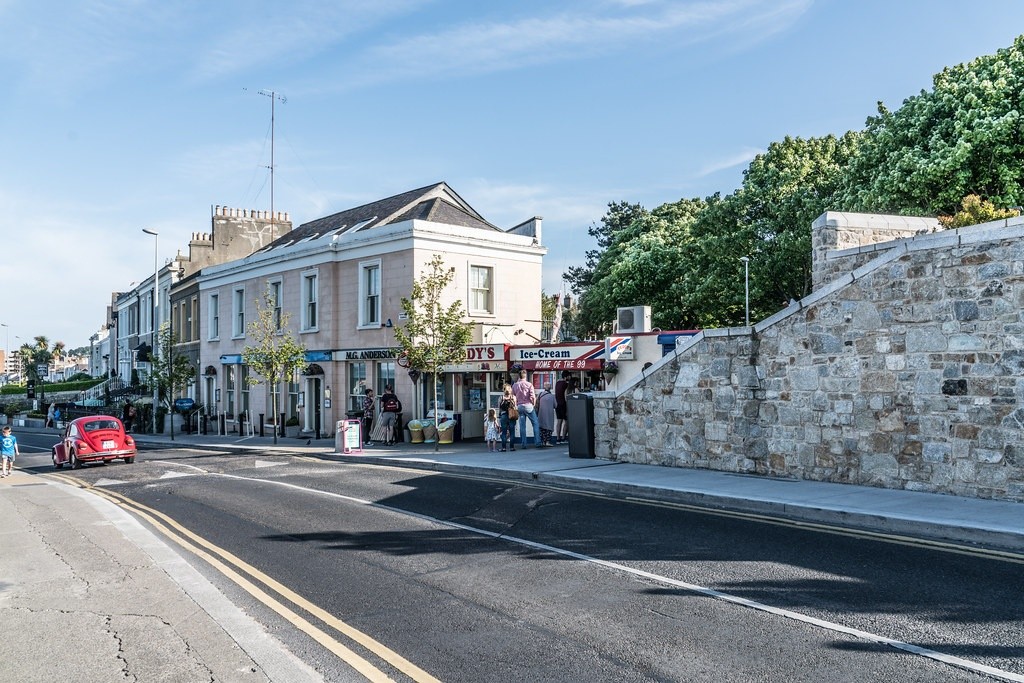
[508,396,518,420]
[535,392,542,417]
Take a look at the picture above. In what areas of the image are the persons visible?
[122,399,135,433]
[0,426,20,478]
[484,369,584,452]
[45,399,56,427]
[360,388,375,447]
[66,399,76,412]
[381,384,402,445]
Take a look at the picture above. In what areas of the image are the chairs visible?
[407,419,424,443]
[437,419,456,443]
[421,420,436,442]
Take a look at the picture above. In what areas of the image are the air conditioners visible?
[613,320,617,334]
[617,305,651,333]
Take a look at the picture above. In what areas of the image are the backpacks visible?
[129,406,136,416]
[385,394,397,411]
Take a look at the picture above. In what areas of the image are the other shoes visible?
[556,440,561,444]
[501,448,506,452]
[8,471,11,476]
[1,474,5,477]
[546,443,554,447]
[365,441,374,446]
[560,440,569,443]
[382,440,395,446]
[510,448,516,451]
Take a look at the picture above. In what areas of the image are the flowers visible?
[510,364,523,373]
[602,362,618,373]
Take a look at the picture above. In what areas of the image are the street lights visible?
[740,257,749,326]
[16,336,21,387]
[1,324,9,376]
[143,229,158,436]
[81,389,84,409]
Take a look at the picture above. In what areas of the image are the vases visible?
[510,373,520,383]
[603,372,615,385]
[409,371,421,385]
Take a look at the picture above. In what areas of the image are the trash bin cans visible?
[566,393,595,459]
[345,409,367,442]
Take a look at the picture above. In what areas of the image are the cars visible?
[52,416,137,470]
